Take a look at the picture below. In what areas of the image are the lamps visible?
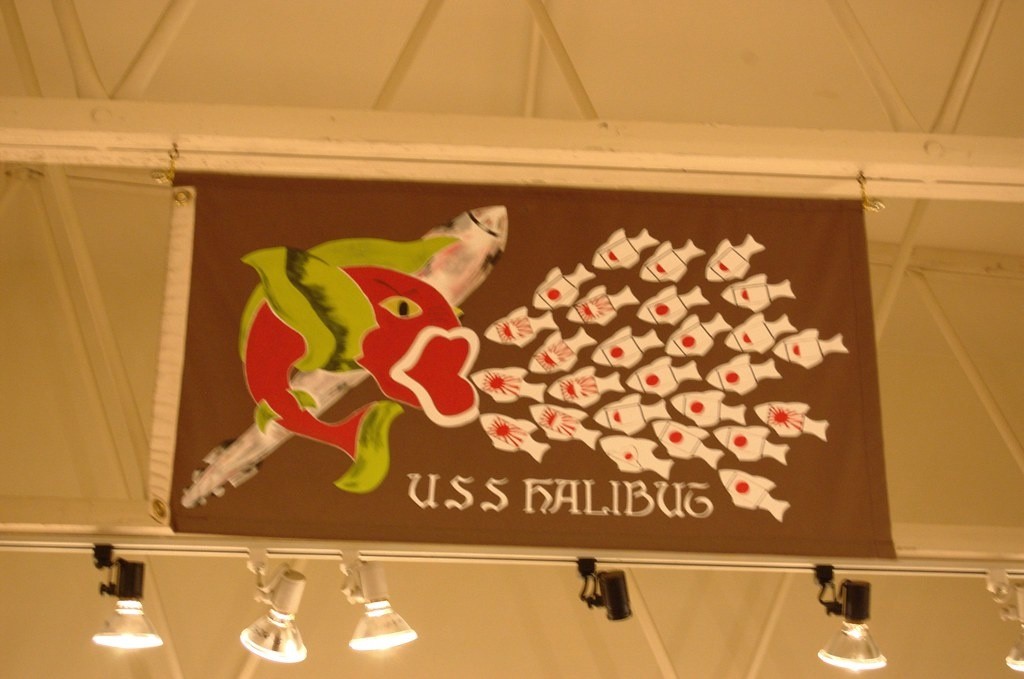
[239,543,306,662]
[986,568,1024,671]
[91,541,162,649]
[813,565,888,670]
[341,558,418,651]
[577,556,632,620]
